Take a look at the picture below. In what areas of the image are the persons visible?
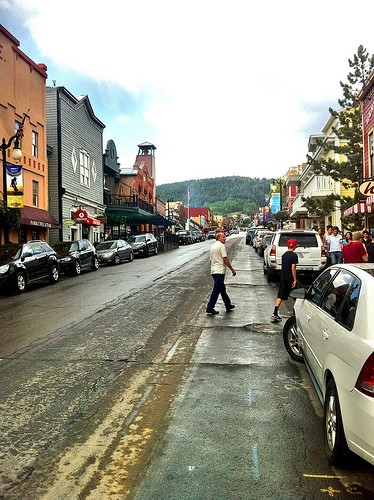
[324,289,337,309]
[313,225,374,269]
[271,240,301,321]
[206,233,235,316]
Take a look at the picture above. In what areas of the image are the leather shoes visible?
[226,304,235,310]
[206,308,219,314]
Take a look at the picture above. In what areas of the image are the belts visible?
[331,251,340,253]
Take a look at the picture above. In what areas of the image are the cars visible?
[246,226,278,258]
[95,239,134,266]
[223,228,240,237]
[282,260,374,464]
[52,238,100,277]
[175,228,216,246]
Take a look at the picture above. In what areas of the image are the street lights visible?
[270,176,284,229]
[0,105,32,243]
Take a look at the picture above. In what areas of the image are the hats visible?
[287,239,300,247]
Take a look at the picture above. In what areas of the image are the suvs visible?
[126,233,159,259]
[263,229,328,284]
[0,239,59,293]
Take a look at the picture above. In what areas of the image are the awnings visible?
[82,216,100,226]
[19,205,60,229]
[344,195,374,218]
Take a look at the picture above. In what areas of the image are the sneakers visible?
[271,314,282,321]
